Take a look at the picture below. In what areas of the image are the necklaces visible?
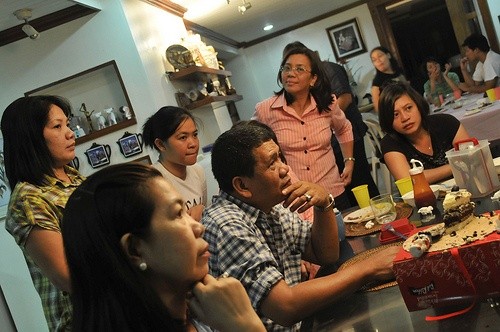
[411,128,430,149]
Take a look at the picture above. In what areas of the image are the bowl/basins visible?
[401,185,441,207]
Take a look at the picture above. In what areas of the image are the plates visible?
[343,202,392,223]
[166,44,192,69]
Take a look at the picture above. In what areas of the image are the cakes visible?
[443,186,475,235]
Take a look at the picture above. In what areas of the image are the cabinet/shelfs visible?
[169,66,243,112]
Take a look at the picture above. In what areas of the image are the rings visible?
[303,193,311,201]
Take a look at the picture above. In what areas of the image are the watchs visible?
[314,192,335,212]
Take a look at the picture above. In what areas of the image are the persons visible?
[422,56,462,104]
[251,49,357,211]
[378,79,472,193]
[282,40,380,206]
[3,95,86,331]
[61,163,269,330]
[456,32,500,93]
[371,46,410,112]
[200,120,410,331]
[140,106,206,222]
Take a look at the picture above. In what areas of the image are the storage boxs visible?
[391,210,500,313]
[445,138,500,198]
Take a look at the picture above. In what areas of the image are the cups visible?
[351,184,370,208]
[486,89,496,103]
[394,177,414,205]
[368,193,397,224]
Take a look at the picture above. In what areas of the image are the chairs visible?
[362,118,395,194]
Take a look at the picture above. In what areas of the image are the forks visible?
[384,224,407,240]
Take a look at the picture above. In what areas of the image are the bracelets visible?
[343,156,355,162]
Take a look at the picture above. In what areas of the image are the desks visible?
[299,174,500,331]
[431,91,500,142]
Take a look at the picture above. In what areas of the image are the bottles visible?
[408,159,437,213]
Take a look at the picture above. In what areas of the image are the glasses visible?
[280,65,313,74]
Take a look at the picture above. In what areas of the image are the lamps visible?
[238,0,252,15]
[12,8,40,39]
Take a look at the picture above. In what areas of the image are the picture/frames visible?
[83,142,111,169]
[325,17,368,62]
[116,130,144,157]
